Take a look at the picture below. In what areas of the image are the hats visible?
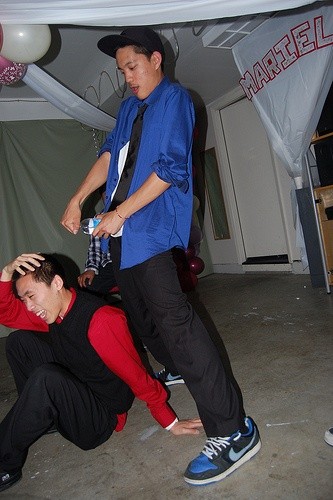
[97,27,166,61]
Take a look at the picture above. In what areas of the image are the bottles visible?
[79,218,102,235]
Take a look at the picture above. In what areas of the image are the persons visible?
[0,252,203,492]
[78,182,119,297]
[61,26,262,485]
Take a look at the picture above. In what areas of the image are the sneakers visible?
[152,368,188,385]
[184,417,262,485]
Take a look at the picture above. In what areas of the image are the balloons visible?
[174,246,204,291]
[0,25,52,86]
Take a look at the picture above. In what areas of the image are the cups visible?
[294,177,303,189]
